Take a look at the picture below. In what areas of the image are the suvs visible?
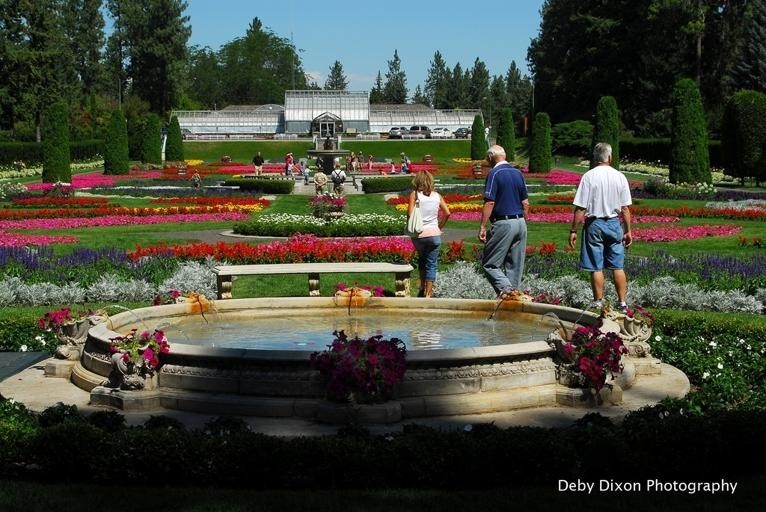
[388,126,409,138]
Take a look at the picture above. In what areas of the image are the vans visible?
[409,124,432,139]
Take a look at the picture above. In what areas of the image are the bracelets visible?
[569,229,578,235]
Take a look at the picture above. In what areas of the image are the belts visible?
[489,214,523,222]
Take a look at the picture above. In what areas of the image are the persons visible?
[191,169,201,188]
[406,169,452,298]
[251,150,412,195]
[568,141,633,316]
[476,143,530,300]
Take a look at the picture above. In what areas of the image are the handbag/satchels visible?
[404,199,424,238]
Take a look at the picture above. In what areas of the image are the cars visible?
[179,127,194,138]
[160,127,168,137]
[454,127,471,138]
[429,127,452,138]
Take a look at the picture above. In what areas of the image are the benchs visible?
[202,185,239,194]
[210,262,413,300]
